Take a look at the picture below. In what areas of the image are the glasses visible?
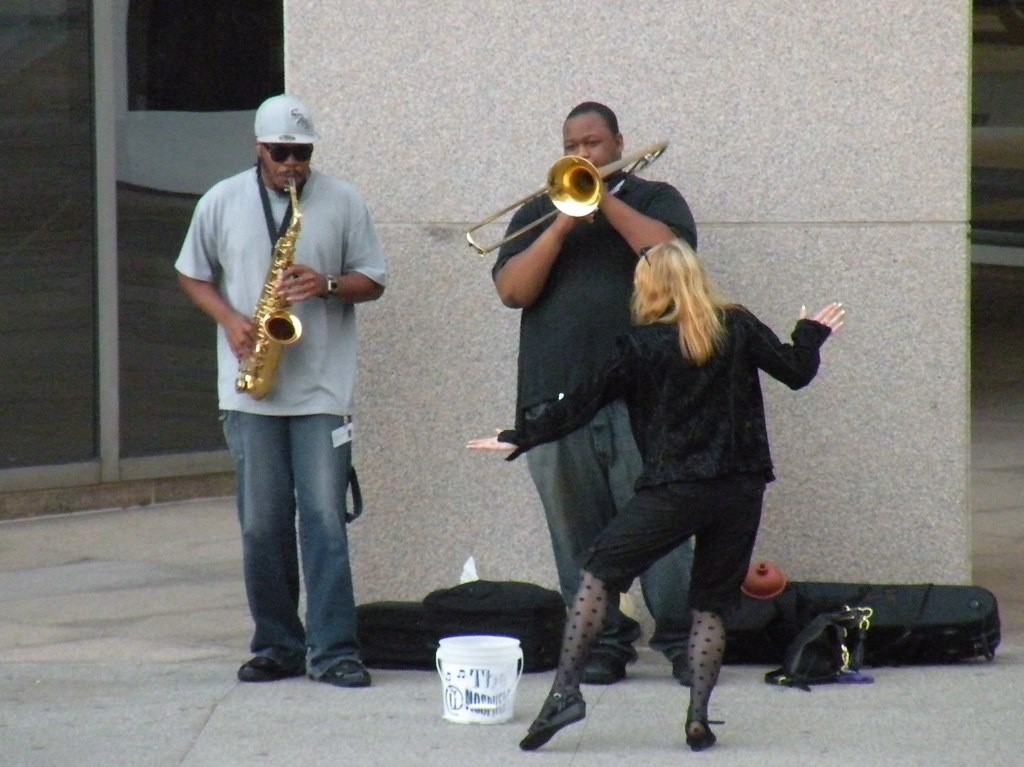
[262,142,314,162]
[638,247,652,271]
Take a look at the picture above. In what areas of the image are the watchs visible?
[320,276,338,299]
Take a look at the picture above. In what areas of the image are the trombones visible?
[463,136,674,257]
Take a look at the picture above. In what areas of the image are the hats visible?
[253,94,323,144]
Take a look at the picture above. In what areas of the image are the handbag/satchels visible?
[765,605,874,692]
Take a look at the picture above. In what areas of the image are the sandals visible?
[519,690,586,749]
[684,717,726,752]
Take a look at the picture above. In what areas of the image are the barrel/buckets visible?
[435,634,523,726]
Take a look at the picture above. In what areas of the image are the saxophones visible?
[231,169,305,402]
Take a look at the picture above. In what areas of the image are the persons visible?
[492,102,697,688]
[465,238,845,752]
[174,96,385,687]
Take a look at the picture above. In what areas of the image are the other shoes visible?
[580,653,627,685]
[238,656,306,681]
[671,654,691,688]
[319,659,370,689]
